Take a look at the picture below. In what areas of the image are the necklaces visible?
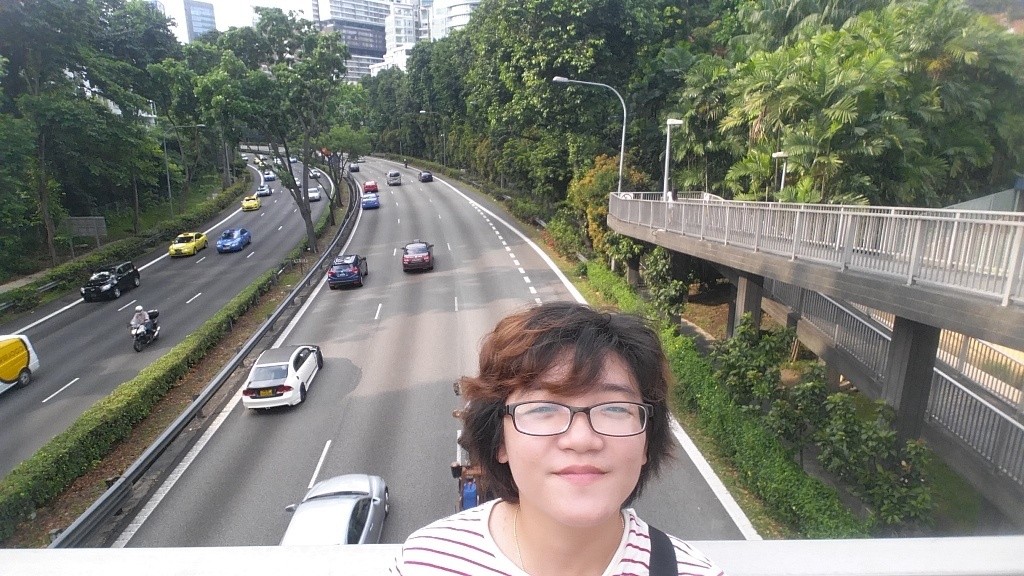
[514,504,524,572]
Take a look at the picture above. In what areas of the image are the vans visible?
[0,335,41,395]
[384,170,401,186]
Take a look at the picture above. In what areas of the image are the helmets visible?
[134,305,144,314]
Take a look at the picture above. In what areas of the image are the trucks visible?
[450,382,500,512]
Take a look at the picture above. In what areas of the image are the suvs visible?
[79,259,140,302]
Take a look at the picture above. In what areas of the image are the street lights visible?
[771,151,790,203]
[663,118,683,201]
[552,75,627,197]
[163,124,206,221]
[419,111,446,167]
[470,101,504,191]
[380,113,402,156]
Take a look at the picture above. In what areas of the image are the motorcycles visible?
[125,309,160,352]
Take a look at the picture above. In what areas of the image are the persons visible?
[377,300,721,576]
[129,304,152,344]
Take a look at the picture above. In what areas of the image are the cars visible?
[168,231,209,257]
[308,188,321,202]
[361,192,380,210]
[363,180,379,194]
[400,241,435,273]
[240,143,365,188]
[215,227,252,254]
[277,473,390,544]
[417,171,433,182]
[257,184,272,197]
[240,195,261,211]
[327,255,368,290]
[241,344,323,413]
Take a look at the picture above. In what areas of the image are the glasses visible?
[499,399,655,437]
